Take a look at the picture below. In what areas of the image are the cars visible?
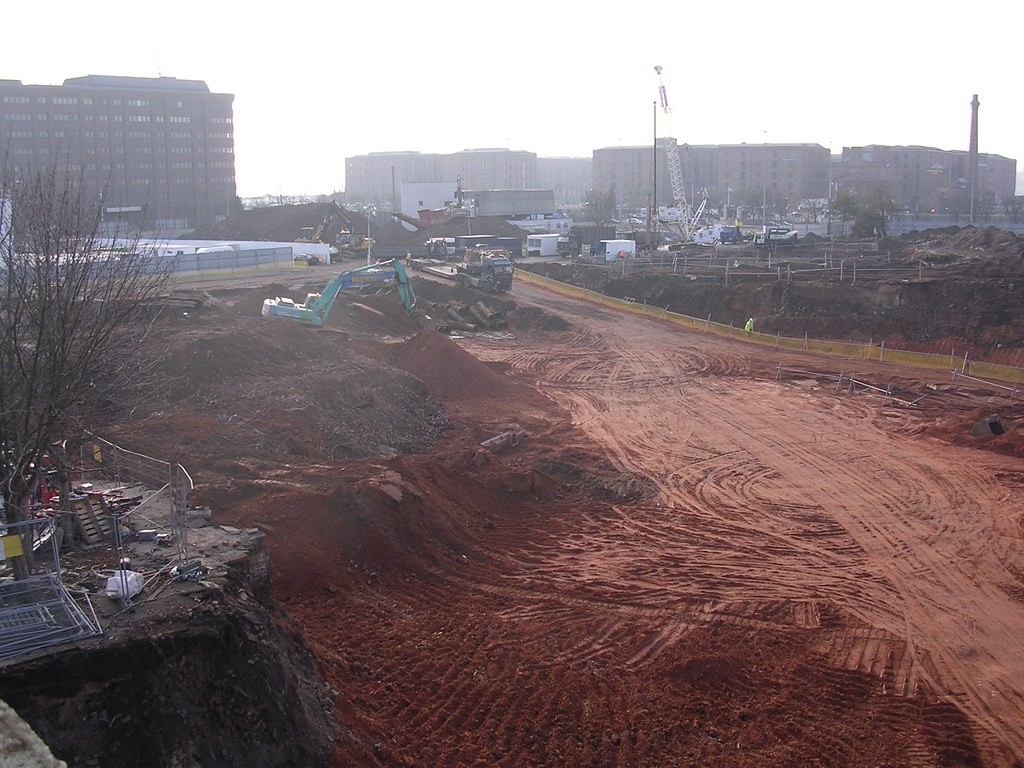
[294,254,320,265]
[599,207,705,223]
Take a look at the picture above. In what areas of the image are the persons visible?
[874,226,877,235]
[620,249,624,258]
[405,249,412,267]
[744,316,754,336]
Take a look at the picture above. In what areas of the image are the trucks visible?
[719,225,754,245]
[13,244,238,269]
[557,225,617,260]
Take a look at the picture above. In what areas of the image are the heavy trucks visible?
[409,252,512,295]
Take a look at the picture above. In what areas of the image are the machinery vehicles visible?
[295,201,353,261]
[261,258,418,340]
[650,65,721,253]
[319,201,375,260]
